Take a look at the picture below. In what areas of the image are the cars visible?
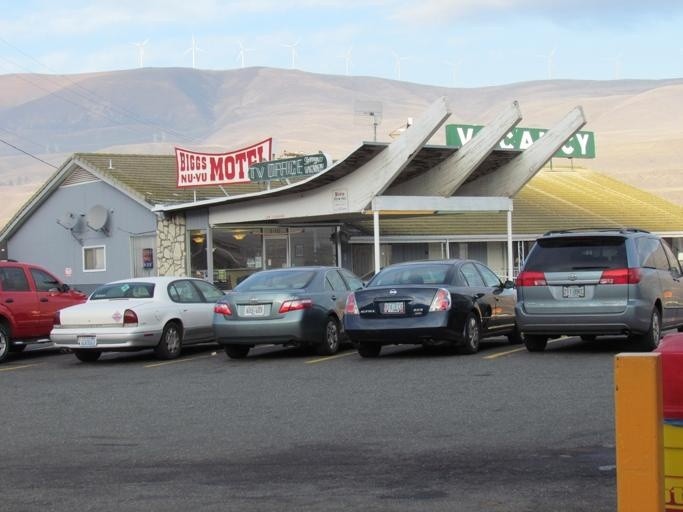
[342,257,519,359]
[48,274,228,361]
[209,264,365,360]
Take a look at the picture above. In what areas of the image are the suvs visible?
[511,226,683,353]
[0,259,88,363]
